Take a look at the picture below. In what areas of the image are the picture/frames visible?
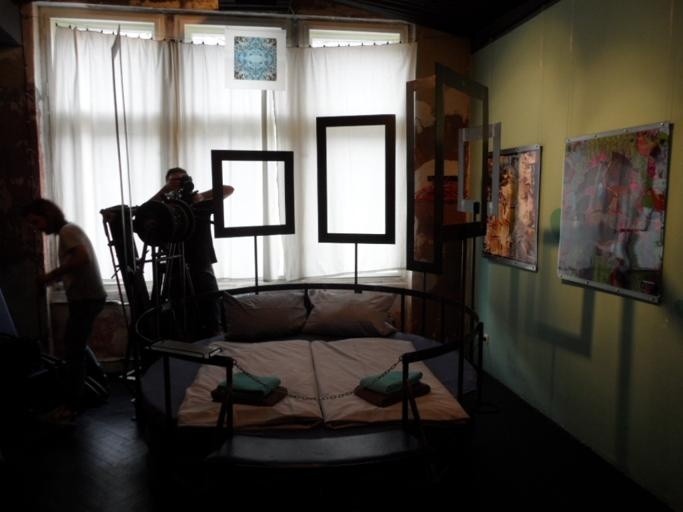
[556,119,672,305]
[479,143,543,272]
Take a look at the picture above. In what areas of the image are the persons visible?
[25,197,106,428]
[144,168,235,337]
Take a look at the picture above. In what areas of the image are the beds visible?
[131,283,486,511]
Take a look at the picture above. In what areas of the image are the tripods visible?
[119,234,200,394]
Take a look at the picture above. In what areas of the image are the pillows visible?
[218,289,396,342]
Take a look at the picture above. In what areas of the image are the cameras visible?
[178,175,194,191]
